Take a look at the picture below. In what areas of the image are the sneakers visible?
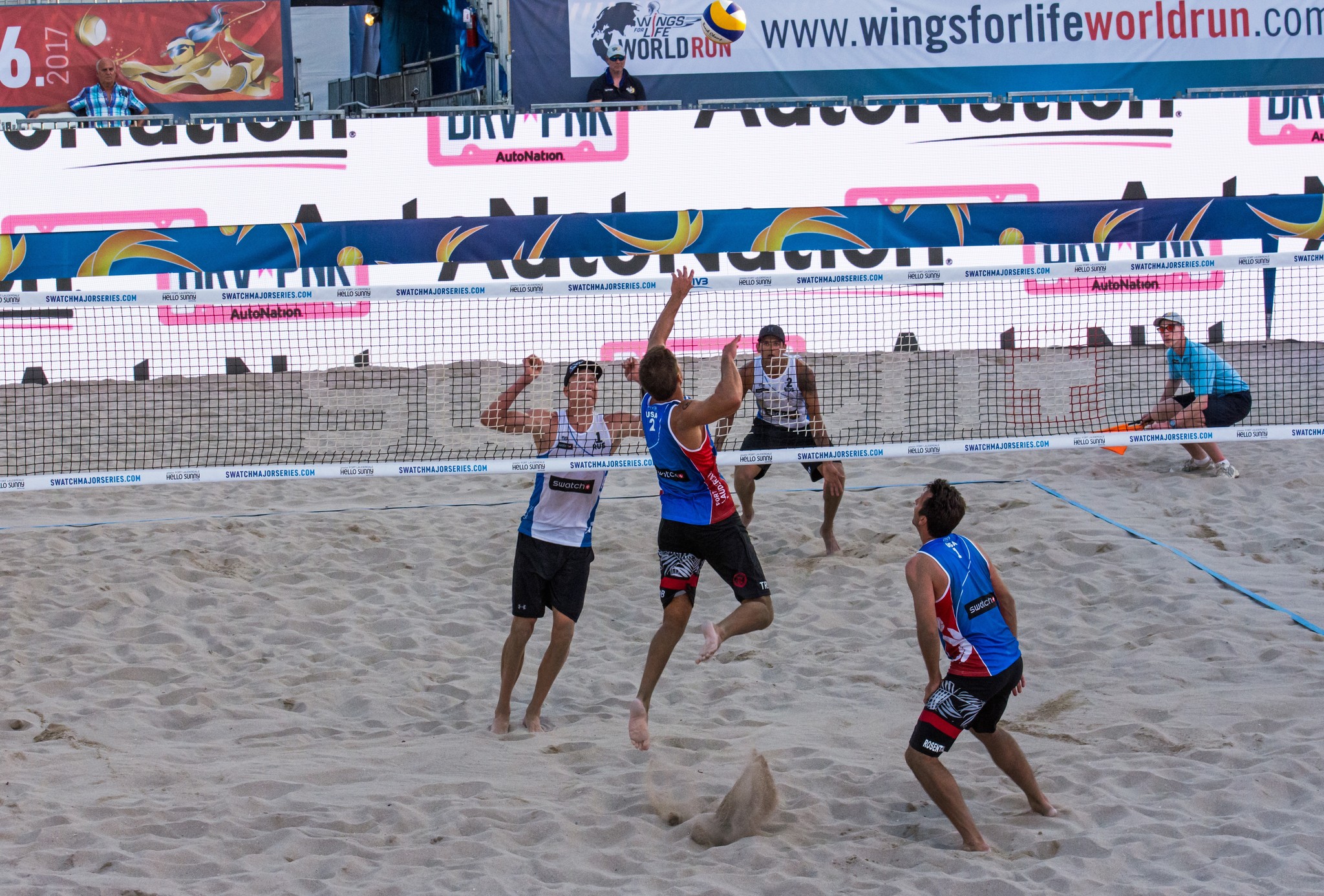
[1169,457,1215,472]
[1214,463,1240,479]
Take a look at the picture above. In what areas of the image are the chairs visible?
[0,110,27,130]
[30,111,78,130]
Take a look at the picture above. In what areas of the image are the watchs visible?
[1168,419,1177,429]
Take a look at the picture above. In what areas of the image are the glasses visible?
[610,56,624,61]
[1156,324,1179,333]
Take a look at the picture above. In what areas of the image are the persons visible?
[28,57,150,128]
[587,45,648,111]
[1139,312,1253,479]
[629,266,774,750]
[480,355,645,735]
[905,478,1058,851]
[713,325,846,556]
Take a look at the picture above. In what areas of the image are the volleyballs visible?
[701,0,746,45]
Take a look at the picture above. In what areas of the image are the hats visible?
[758,323,786,343]
[607,44,625,58]
[1153,312,1185,327]
[564,359,602,387]
